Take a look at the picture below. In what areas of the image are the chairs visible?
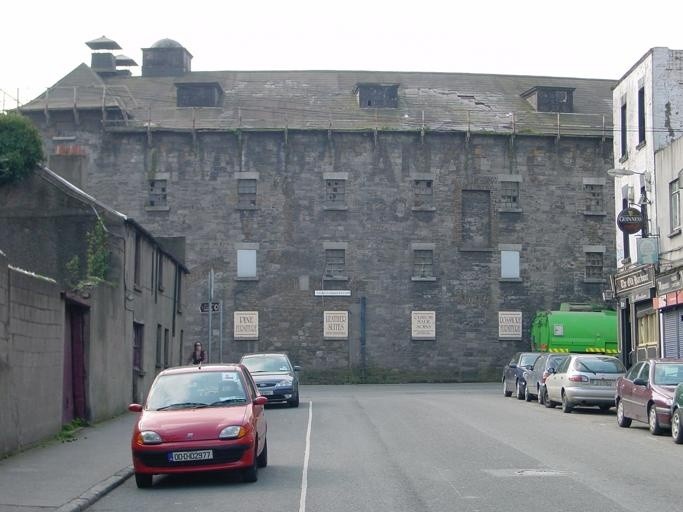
[211,380,241,402]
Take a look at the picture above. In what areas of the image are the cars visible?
[125,351,301,490]
[499,350,683,446]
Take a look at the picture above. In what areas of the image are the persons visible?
[186,341,208,366]
